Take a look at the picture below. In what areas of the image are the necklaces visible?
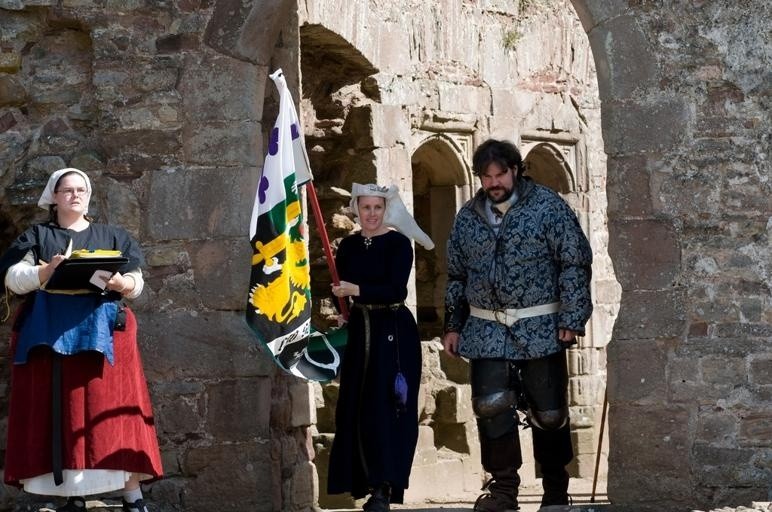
[361,228,379,250]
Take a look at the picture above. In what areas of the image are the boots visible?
[476,478,521,510]
[539,476,569,511]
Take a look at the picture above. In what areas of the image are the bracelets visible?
[116,280,128,293]
[349,283,354,296]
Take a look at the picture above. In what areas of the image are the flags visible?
[239,67,350,384]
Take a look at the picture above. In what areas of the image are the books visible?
[39,249,130,296]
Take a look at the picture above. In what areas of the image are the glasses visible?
[54,187,86,196]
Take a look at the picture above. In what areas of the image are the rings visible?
[339,281,345,298]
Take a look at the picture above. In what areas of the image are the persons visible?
[440,138,595,512]
[318,180,437,512]
[0,166,167,512]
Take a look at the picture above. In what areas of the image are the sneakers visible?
[362,493,391,511]
